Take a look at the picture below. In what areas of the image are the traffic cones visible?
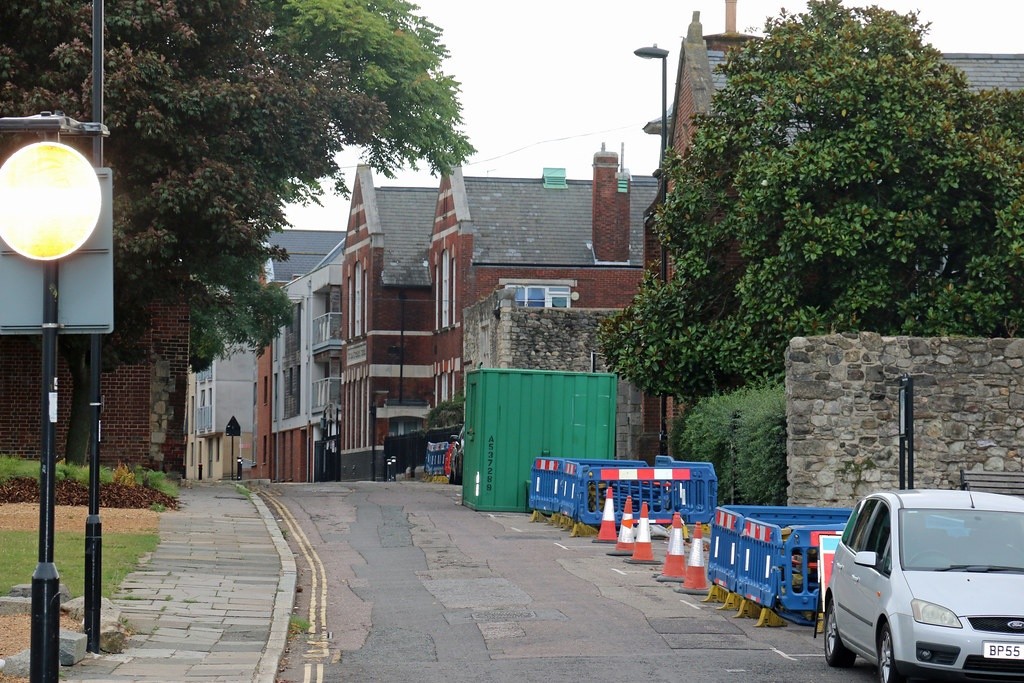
[673,522,710,594]
[606,496,635,556]
[656,512,686,582]
[623,502,664,565]
[592,487,618,543]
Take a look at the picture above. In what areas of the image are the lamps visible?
[423,258,434,287]
[572,280,579,302]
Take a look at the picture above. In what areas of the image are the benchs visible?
[960,469,1024,498]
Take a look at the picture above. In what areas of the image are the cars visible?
[824,483,1024,683]
[449,424,465,485]
